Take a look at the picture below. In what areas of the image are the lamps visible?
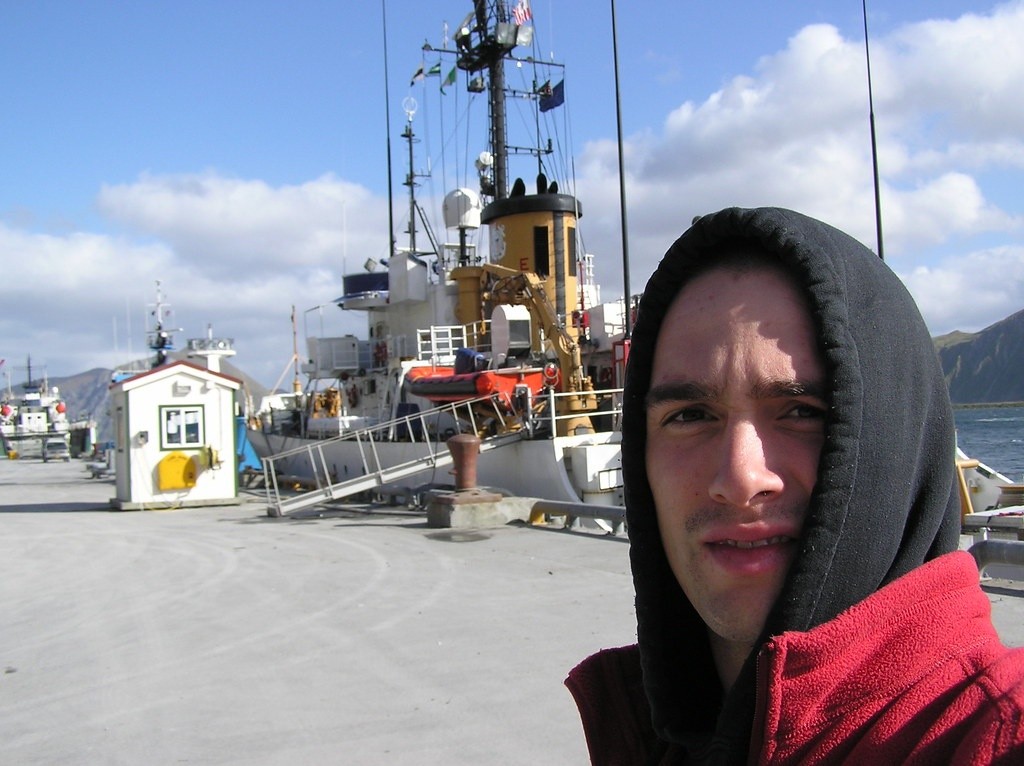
[496,22,534,47]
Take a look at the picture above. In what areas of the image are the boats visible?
[89,278,264,474]
[405,346,553,416]
[0,353,100,456]
[244,0,644,534]
[858,0,1024,543]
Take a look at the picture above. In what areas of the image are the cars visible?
[42,437,72,463]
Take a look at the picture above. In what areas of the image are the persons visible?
[564,205,1024,766]
